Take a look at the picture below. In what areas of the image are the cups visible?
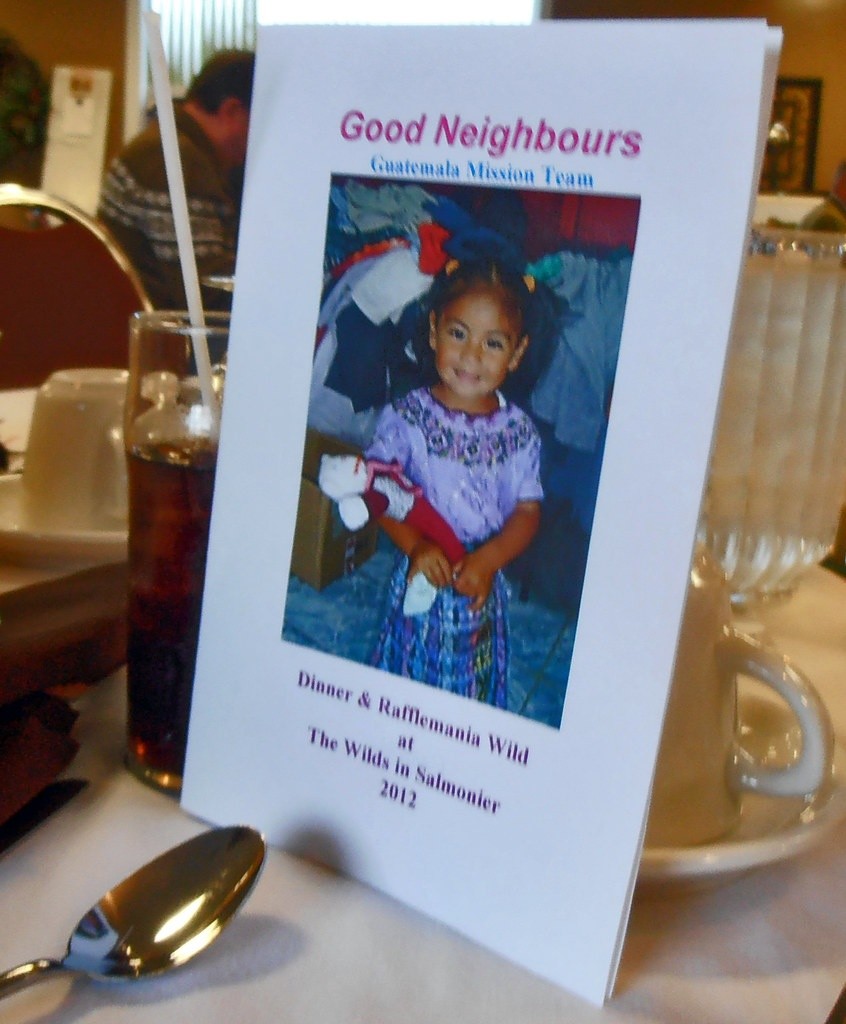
[651,564,832,842]
[124,312,224,792]
[18,369,127,529]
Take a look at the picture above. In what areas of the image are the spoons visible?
[0,825,265,989]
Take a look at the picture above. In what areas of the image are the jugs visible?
[686,232,846,599]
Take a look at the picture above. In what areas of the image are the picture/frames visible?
[758,76,823,193]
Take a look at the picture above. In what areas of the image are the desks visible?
[0,561,846,1024]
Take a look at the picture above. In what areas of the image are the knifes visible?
[0,780,85,851]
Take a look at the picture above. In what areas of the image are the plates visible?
[634,685,846,873]
[0,475,129,564]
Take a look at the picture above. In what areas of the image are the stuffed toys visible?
[319,454,471,617]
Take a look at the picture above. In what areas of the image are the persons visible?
[354,239,568,717]
[100,47,253,374]
